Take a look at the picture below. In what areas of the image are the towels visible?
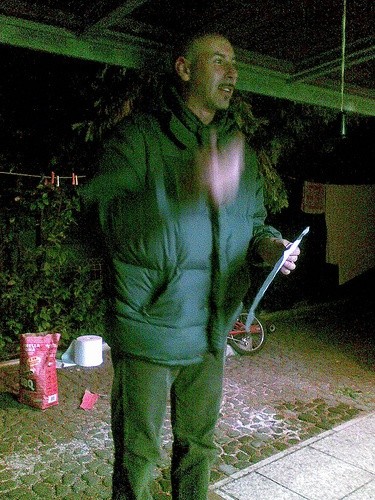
[300,179,325,214]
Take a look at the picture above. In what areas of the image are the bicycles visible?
[227,311,276,356]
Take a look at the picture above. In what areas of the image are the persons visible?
[75,28,301,500]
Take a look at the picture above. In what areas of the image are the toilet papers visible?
[61,334,103,368]
